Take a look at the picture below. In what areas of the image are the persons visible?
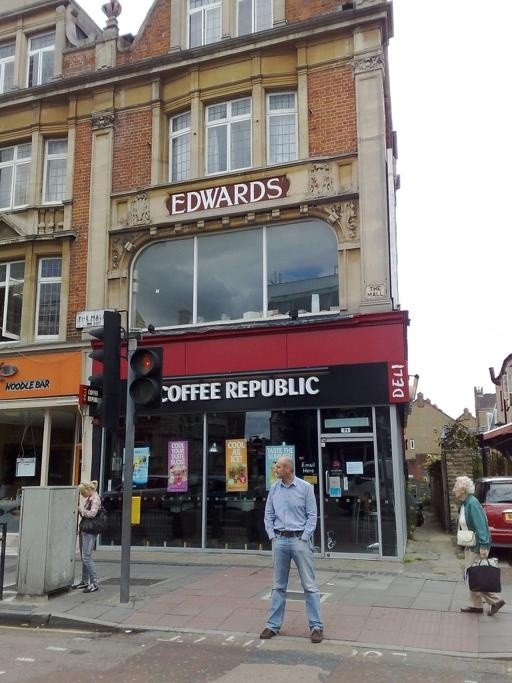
[452,476,506,615]
[73,479,101,592]
[259,455,326,644]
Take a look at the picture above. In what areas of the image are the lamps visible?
[209,443,218,453]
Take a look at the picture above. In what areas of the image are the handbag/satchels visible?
[465,560,505,591]
[79,507,108,533]
[456,530,476,547]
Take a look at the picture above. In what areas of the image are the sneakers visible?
[71,581,99,593]
[311,629,323,643]
[461,600,505,617]
[259,628,276,639]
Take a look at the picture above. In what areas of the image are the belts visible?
[274,530,304,536]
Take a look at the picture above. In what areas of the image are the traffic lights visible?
[129,348,164,410]
[89,327,104,389]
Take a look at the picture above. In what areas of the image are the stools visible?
[363,511,378,544]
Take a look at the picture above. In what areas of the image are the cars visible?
[342,458,393,512]
[474,476,512,549]
[103,474,241,512]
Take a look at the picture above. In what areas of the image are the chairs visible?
[102,508,266,551]
